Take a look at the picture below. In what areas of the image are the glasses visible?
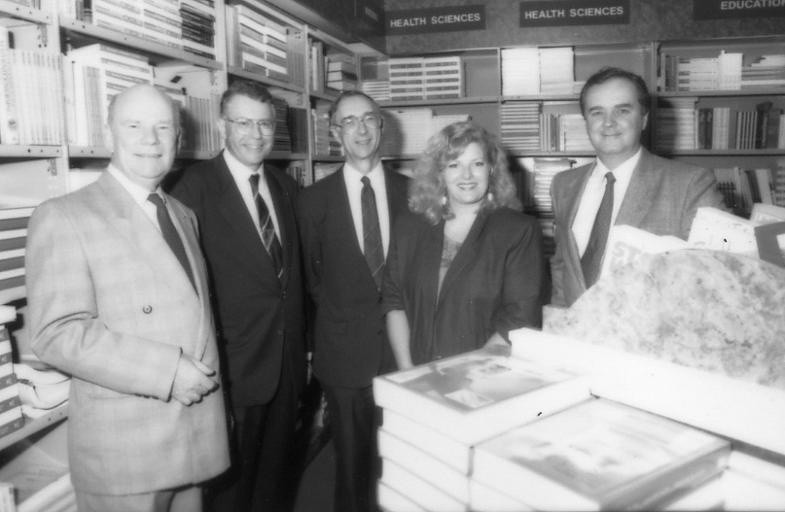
[223,116,277,137]
[332,114,384,130]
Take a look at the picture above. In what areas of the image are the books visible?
[226,1,784,267]
[369,305,785,512]
[2,1,227,266]
[0,267,80,510]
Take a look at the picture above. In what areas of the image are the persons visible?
[375,120,549,371]
[296,89,419,512]
[549,68,734,308]
[168,82,318,511]
[25,83,233,511]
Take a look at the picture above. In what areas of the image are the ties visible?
[359,175,386,296]
[578,170,617,292]
[146,192,202,299]
[248,172,287,302]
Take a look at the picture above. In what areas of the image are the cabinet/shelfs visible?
[370,36,783,198]
[2,0,376,512]
[369,201,782,511]
